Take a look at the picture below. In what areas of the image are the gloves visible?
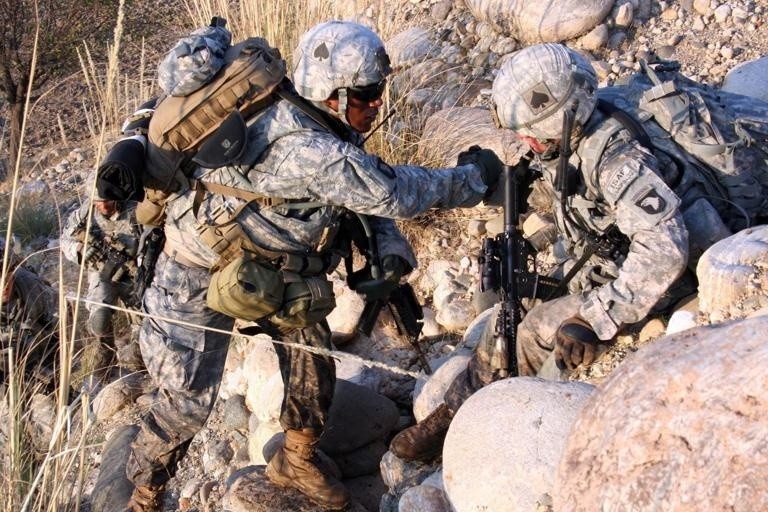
[553,313,600,370]
[80,245,103,266]
[357,257,411,301]
[458,145,504,199]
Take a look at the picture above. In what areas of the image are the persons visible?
[389,41,733,464]
[123,19,505,511]
[1,238,74,400]
[60,169,149,377]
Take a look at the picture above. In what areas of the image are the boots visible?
[266,428,352,509]
[127,484,164,512]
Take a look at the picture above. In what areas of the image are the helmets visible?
[491,43,598,141]
[0,238,17,273]
[293,21,393,102]
[86,168,110,200]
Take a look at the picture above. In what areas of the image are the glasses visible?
[336,80,387,101]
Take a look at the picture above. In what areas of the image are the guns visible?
[70,222,144,305]
[335,206,434,375]
[481,165,567,384]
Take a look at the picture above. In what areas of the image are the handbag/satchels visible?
[158,18,231,97]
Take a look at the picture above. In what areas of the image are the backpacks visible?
[133,34,285,229]
[625,48,768,232]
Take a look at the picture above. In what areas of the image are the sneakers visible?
[390,404,453,462]
